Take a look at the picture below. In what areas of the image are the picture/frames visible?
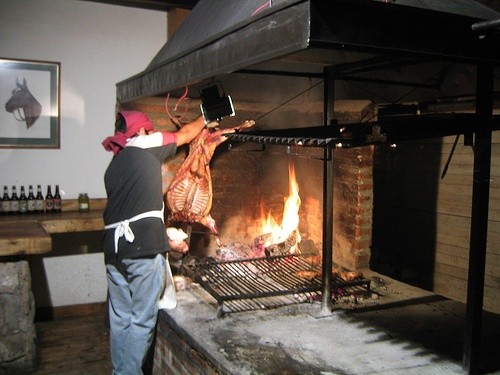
[0,57,62,149]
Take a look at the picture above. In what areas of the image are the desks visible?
[0,199,110,375]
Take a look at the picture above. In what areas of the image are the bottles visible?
[78,193,89,212]
[53,185,61,214]
[19,186,27,214]
[36,185,44,214]
[11,186,19,214]
[27,185,36,213]
[1,186,11,214]
[46,185,53,214]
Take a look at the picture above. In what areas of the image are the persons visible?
[104,111,220,375]
